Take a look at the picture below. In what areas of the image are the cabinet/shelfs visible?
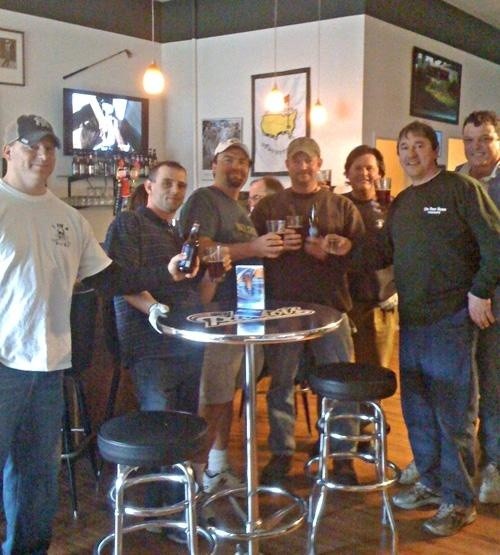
[64,171,148,209]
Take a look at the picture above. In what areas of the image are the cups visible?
[266,219,286,263]
[85,186,114,197]
[286,215,306,255]
[60,195,115,206]
[317,169,332,185]
[204,246,225,284]
[374,177,391,212]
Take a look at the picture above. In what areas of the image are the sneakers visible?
[201,469,248,495]
[331,455,360,488]
[396,462,424,485]
[421,498,478,538]
[390,479,443,510]
[260,452,293,486]
[358,424,380,453]
[142,507,187,545]
[478,463,500,504]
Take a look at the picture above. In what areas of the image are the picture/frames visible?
[410,45,462,125]
[197,117,243,181]
[0,27,25,88]
[249,66,310,176]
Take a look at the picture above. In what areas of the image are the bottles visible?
[308,202,319,238]
[71,148,158,176]
[177,220,201,273]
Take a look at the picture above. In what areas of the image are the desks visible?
[157,299,345,554]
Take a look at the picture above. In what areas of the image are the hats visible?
[286,136,321,164]
[3,113,62,150]
[214,137,251,161]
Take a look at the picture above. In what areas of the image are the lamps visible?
[265,0,285,114]
[142,0,165,95]
[310,0,327,126]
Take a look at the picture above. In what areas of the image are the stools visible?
[304,362,402,554]
[93,410,219,554]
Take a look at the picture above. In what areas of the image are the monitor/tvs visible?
[62,87,151,160]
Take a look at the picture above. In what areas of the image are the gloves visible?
[146,302,170,335]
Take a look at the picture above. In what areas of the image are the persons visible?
[322,121,499,537]
[247,178,283,211]
[398,110,499,504]
[313,145,400,456]
[178,138,286,500]
[0,114,112,554]
[129,182,150,212]
[251,136,366,488]
[105,162,223,544]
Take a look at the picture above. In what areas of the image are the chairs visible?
[60,289,98,520]
[103,292,122,420]
